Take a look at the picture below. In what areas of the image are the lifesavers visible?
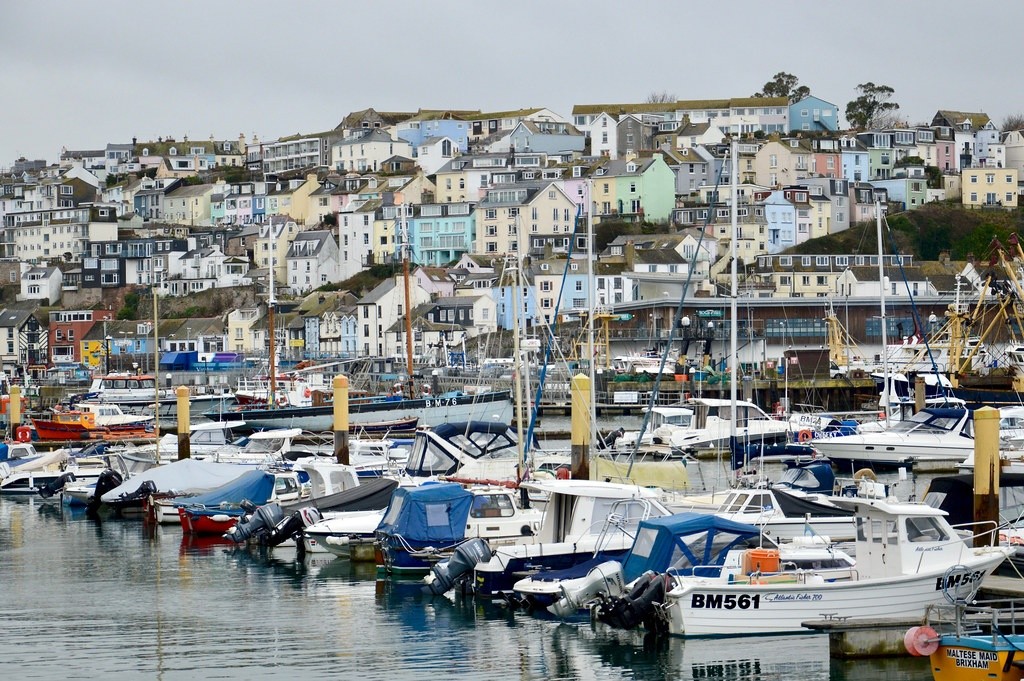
[799,429,811,443]
[276,395,287,406]
[854,469,877,487]
[305,388,311,397]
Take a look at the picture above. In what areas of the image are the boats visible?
[0,128,1024,680]
